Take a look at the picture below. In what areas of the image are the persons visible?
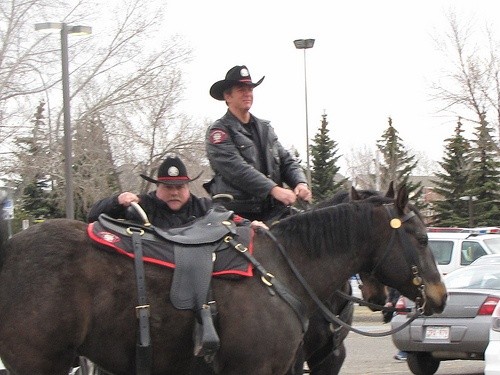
[203,65,313,222]
[383,284,410,361]
[88,158,268,230]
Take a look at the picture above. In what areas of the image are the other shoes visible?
[394,352,407,361]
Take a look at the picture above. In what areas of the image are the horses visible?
[2,180,448,375]
[286,181,404,373]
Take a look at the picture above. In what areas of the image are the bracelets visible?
[298,182,310,189]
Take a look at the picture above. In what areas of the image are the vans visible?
[426,225,500,278]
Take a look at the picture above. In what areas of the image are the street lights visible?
[293,38,318,208]
[34,21,93,219]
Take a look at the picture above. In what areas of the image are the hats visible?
[139,156,204,185]
[210,65,265,100]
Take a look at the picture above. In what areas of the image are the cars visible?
[389,253,500,375]
